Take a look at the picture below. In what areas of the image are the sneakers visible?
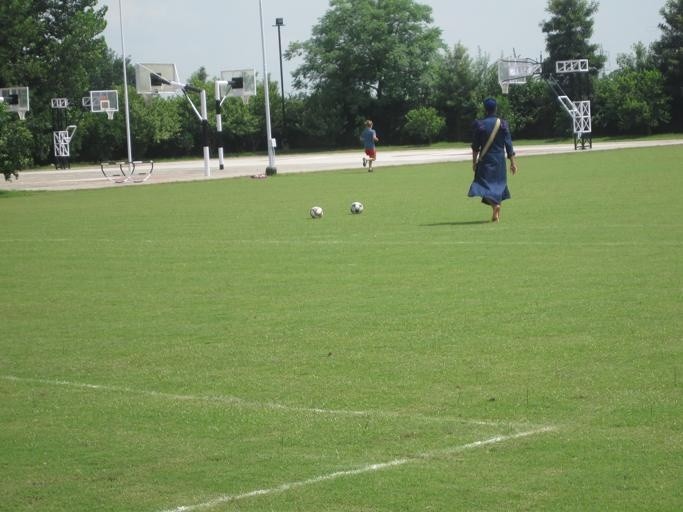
[368,168,373,172]
[363,158,366,166]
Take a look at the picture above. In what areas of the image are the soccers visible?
[310,206,323,219]
[350,202,363,214]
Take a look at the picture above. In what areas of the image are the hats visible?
[484,97,496,112]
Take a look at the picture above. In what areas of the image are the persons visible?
[466,98,517,223]
[358,119,379,172]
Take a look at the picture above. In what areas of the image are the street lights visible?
[271,18,287,133]
